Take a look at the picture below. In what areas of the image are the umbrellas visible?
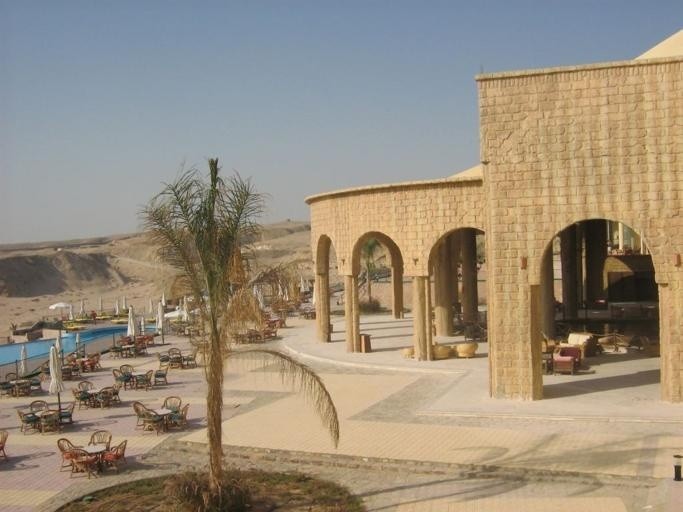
[18,344,28,374]
[49,301,74,315]
[76,332,81,352]
[69,291,196,344]
[49,343,65,422]
[56,335,64,367]
[253,275,310,309]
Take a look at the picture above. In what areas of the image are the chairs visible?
[233,300,316,344]
[0,330,198,481]
[550,343,582,375]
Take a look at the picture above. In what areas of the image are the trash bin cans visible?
[360,333,371,353]
